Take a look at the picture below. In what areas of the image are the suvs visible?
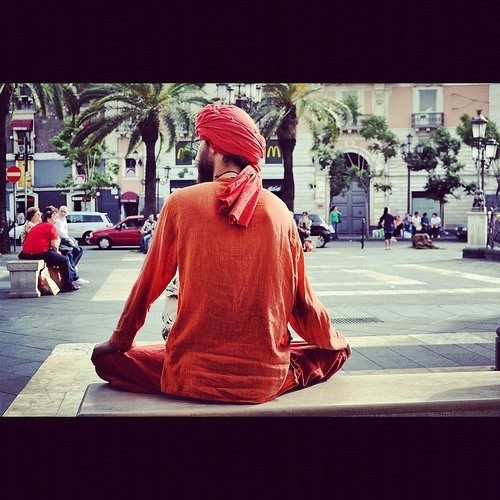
[65,213,114,245]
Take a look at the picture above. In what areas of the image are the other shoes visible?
[74,286,79,290]
[73,278,89,284]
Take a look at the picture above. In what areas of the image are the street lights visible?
[397,132,424,216]
[470,109,499,195]
[156,164,172,214]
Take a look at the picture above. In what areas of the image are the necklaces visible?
[215,170,240,180]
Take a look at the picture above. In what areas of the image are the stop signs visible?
[7,166,21,183]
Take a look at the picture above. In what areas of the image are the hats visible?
[195,104,266,228]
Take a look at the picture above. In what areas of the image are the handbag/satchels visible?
[338,210,342,223]
[38,275,59,296]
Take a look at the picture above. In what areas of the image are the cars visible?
[294,214,330,248]
[85,215,145,248]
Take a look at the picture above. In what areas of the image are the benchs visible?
[6,260,45,298]
[76,369,500,418]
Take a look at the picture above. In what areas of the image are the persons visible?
[378,207,395,251]
[91,104,351,404]
[297,212,312,245]
[17,207,24,225]
[391,212,441,238]
[330,206,342,240]
[19,205,90,291]
[139,211,160,254]
